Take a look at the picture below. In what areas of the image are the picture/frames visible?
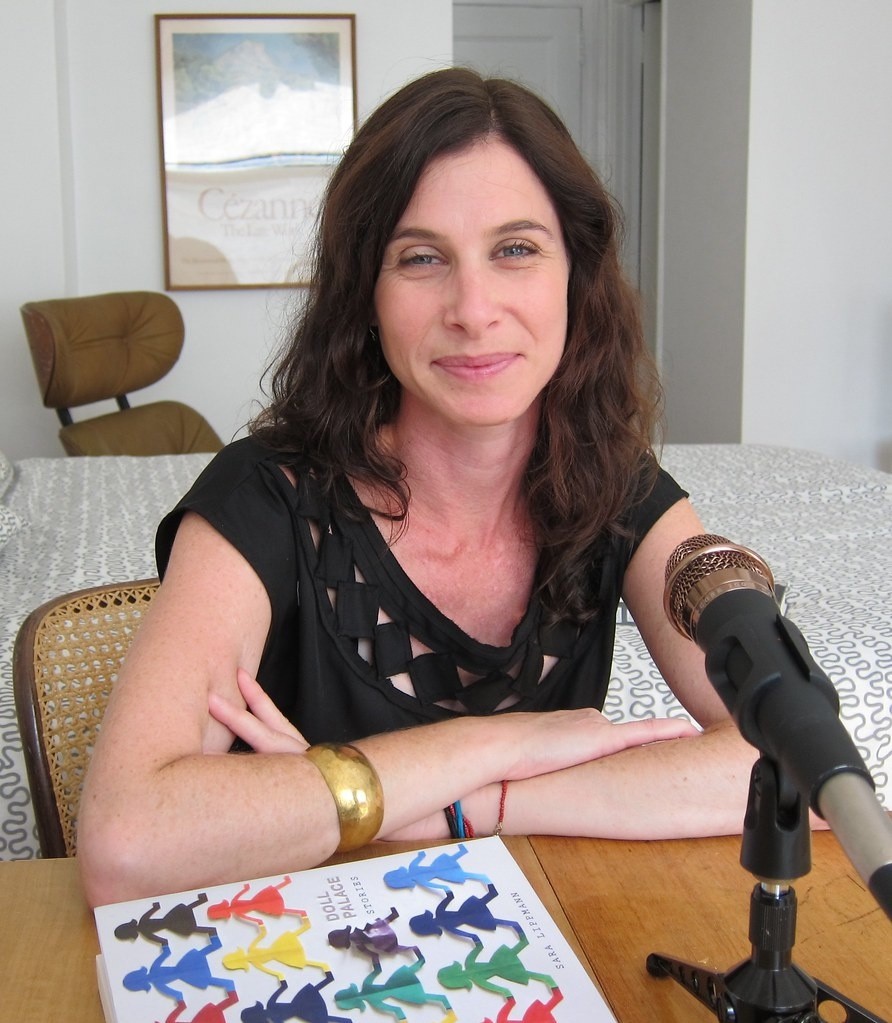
[153,11,361,292]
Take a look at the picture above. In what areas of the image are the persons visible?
[76,66,833,906]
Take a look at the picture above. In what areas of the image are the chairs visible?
[20,290,230,456]
[14,578,184,859]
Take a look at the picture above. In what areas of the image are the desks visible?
[0,830,892,1023]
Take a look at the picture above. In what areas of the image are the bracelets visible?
[307,743,384,852]
[497,780,509,836]
[444,802,474,838]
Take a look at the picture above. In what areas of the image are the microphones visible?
[661,534,892,921]
